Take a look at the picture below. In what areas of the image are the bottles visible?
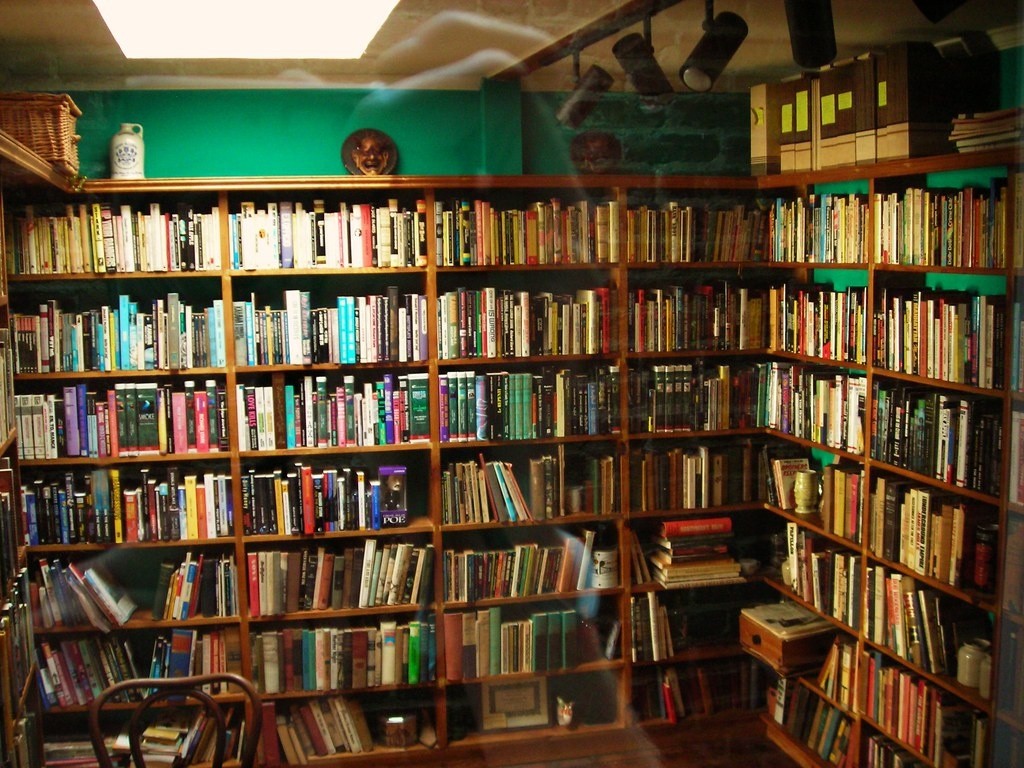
[111,123,144,178]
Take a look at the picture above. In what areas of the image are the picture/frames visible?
[477,676,554,734]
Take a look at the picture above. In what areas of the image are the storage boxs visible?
[740,601,842,678]
[752,41,960,176]
[0,90,82,175]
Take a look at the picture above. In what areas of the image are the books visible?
[751,41,1024,176]
[0,172,1024,768]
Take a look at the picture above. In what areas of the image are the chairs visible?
[89,674,261,768]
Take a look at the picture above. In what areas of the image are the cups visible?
[958,639,991,687]
[794,469,818,514]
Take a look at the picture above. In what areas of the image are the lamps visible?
[680,0,748,93]
[785,0,838,68]
[614,18,676,107]
[556,52,614,132]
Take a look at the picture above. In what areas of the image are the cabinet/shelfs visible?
[0,128,1024,768]
